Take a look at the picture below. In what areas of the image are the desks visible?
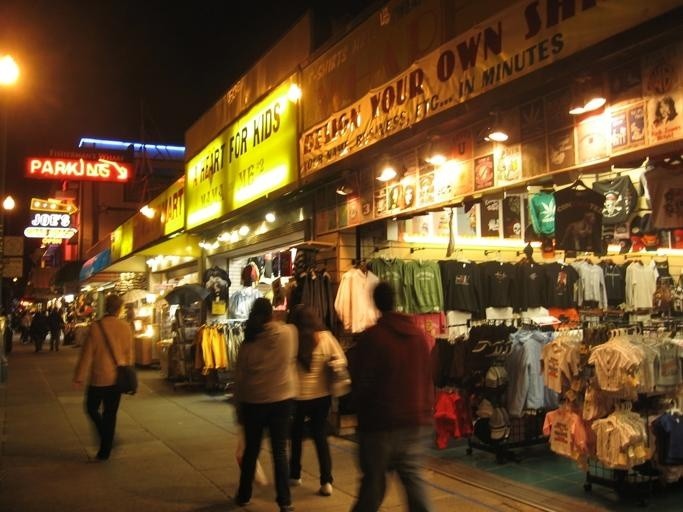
[136,337,152,365]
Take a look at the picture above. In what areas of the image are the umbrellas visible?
[163,283,211,305]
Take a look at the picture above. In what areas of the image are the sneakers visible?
[280,479,301,512]
[236,491,249,504]
[320,482,333,495]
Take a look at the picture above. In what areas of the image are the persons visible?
[387,185,401,211]
[232,297,298,512]
[70,295,136,460]
[0,296,71,375]
[348,281,438,512]
[402,185,415,210]
[650,94,679,126]
[285,306,349,495]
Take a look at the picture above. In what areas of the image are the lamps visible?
[335,60,607,198]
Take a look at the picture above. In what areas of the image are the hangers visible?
[584,252,593,264]
[457,250,471,264]
[668,155,683,165]
[552,326,682,416]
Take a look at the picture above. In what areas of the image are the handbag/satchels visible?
[117,366,137,394]
